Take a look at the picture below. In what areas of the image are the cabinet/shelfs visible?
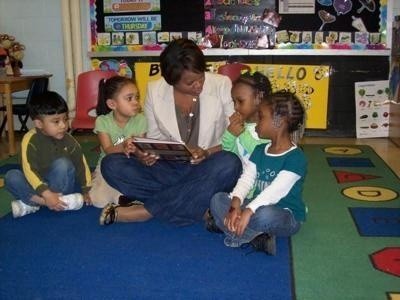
[86,0,393,138]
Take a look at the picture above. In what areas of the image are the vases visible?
[5,63,15,75]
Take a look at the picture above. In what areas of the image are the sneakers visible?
[58,192,85,211]
[10,199,40,219]
[248,232,277,257]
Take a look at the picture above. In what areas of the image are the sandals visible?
[99,200,121,226]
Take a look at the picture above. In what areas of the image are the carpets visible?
[0,144,400,300]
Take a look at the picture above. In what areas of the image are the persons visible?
[99,38,244,234]
[209,91,308,257]
[4,90,93,218]
[221,70,273,199]
[87,75,148,209]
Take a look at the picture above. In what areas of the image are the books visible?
[131,136,196,161]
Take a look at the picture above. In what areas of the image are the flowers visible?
[0,34,25,68]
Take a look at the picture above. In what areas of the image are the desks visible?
[0,73,52,157]
